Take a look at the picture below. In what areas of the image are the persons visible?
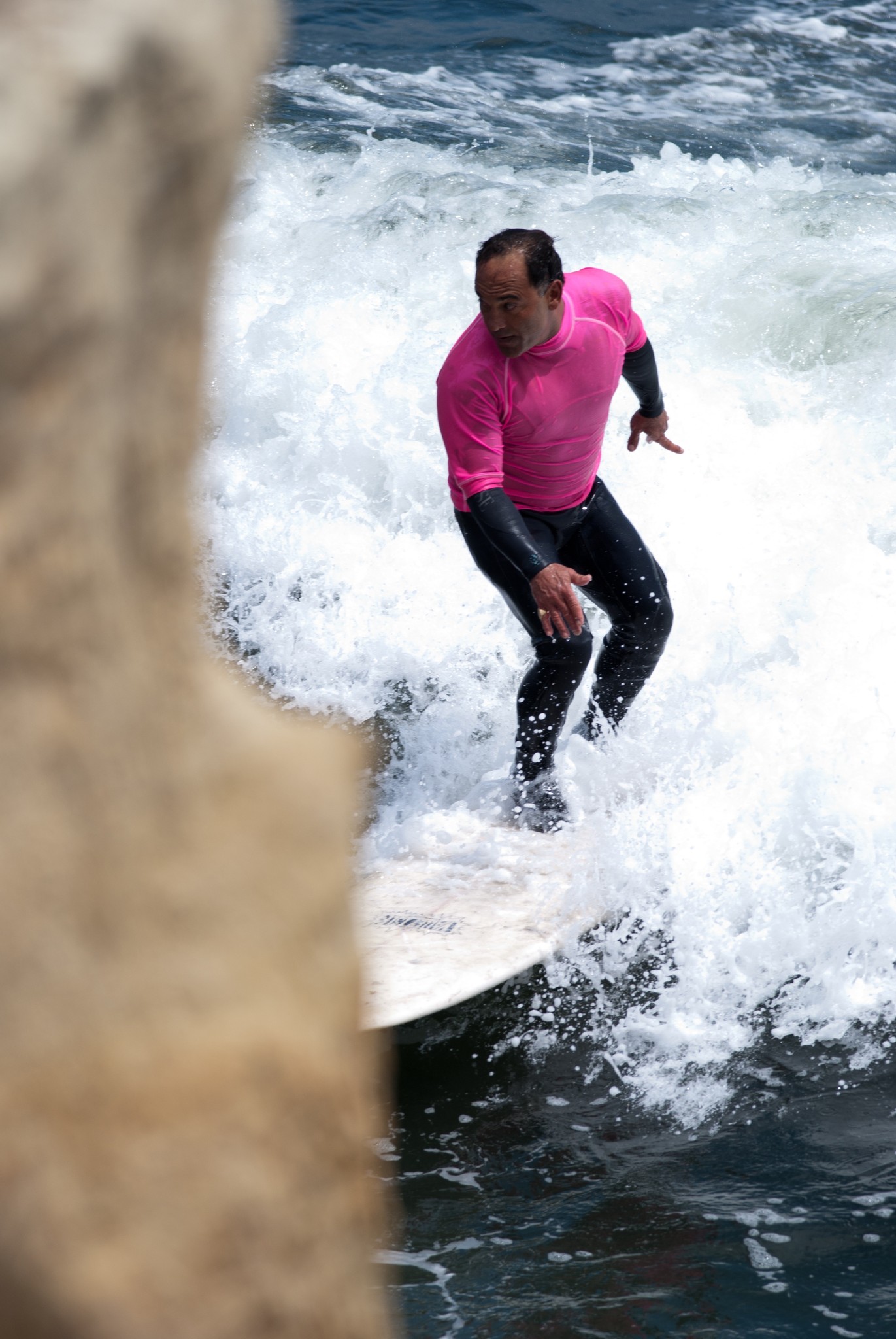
[436,228,683,830]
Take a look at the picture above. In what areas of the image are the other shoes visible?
[568,718,614,749]
[502,765,569,832]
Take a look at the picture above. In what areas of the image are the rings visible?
[537,608,546,619]
